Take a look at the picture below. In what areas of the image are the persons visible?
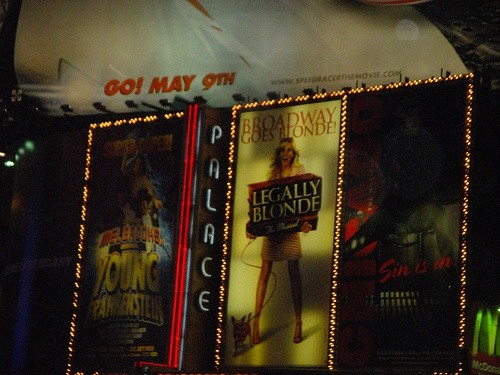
[251,138,313,345]
[381,112,445,265]
[111,145,160,228]
